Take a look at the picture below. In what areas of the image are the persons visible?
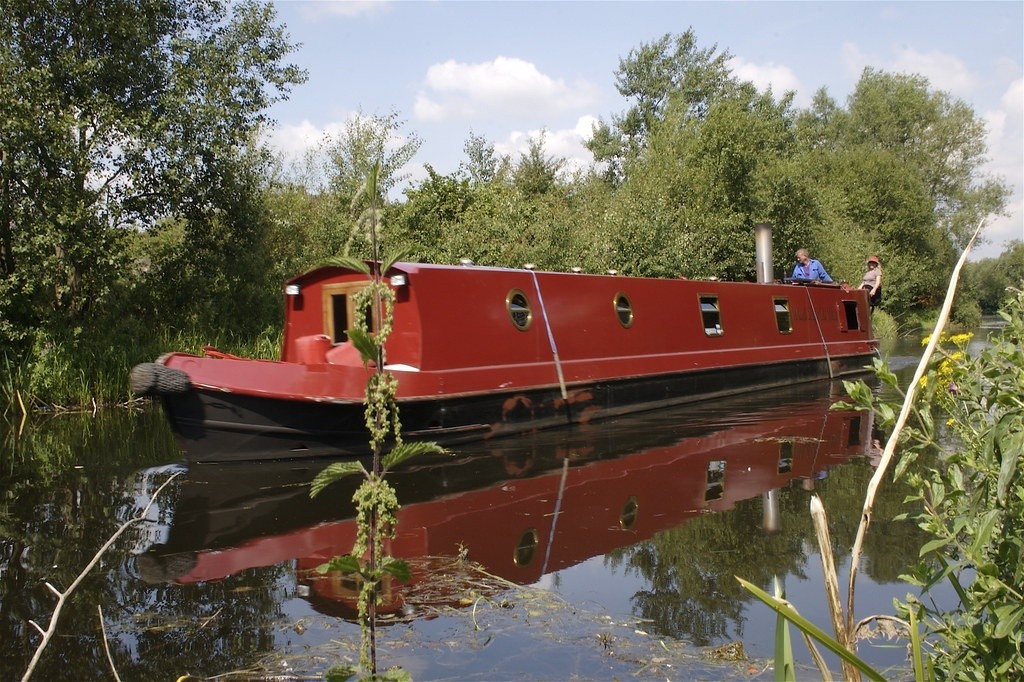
[857,255,882,306]
[791,248,833,284]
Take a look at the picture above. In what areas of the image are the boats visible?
[123,358,880,628]
[131,220,881,435]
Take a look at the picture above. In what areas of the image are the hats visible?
[867,256,879,264]
